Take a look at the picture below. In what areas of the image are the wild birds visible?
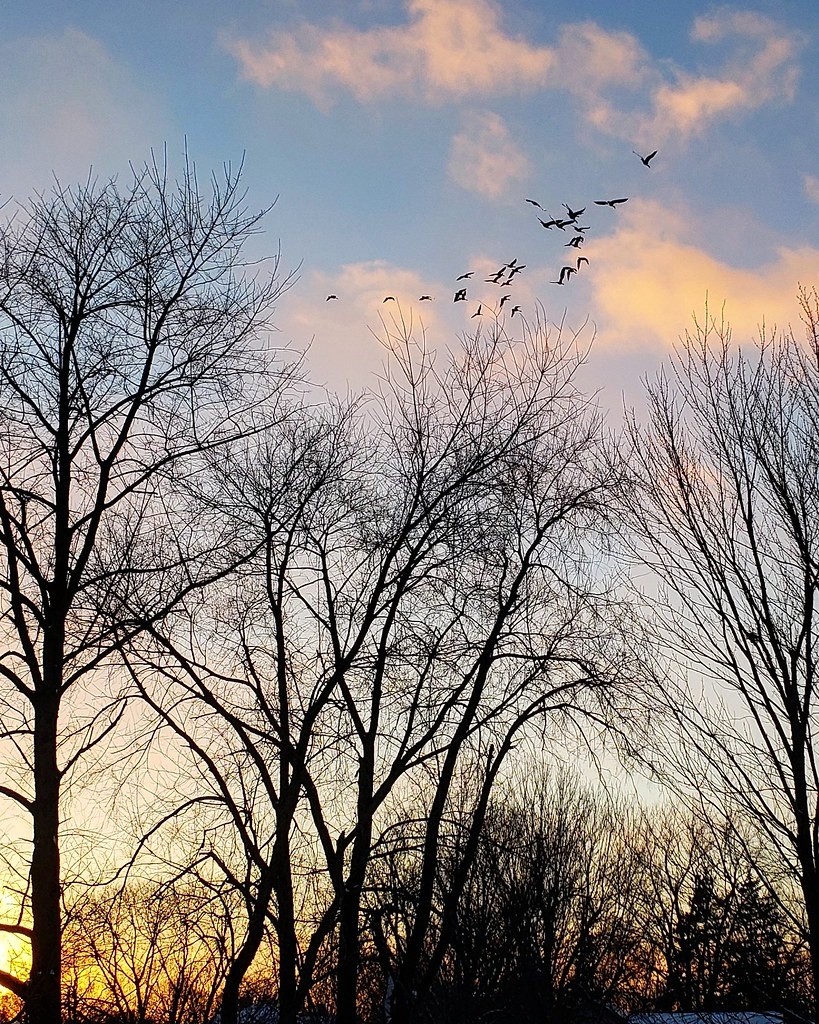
[484,257,527,287]
[382,296,395,303]
[524,197,590,250]
[593,198,630,209]
[453,288,468,303]
[324,294,339,301]
[471,304,483,319]
[511,305,522,318]
[500,294,511,307]
[632,149,658,168]
[549,256,591,286]
[418,295,433,301]
[456,272,475,281]
